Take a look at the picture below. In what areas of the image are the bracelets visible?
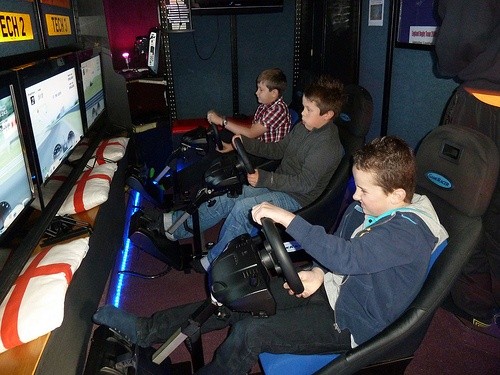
[222,120,227,127]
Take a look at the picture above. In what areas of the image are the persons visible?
[434,0,500,328]
[140,77,345,272]
[150,67,292,183]
[93,136,449,375]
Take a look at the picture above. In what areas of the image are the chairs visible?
[300,84,373,230]
[259,122,500,375]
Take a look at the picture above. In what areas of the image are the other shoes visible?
[130,211,173,247]
[128,175,161,204]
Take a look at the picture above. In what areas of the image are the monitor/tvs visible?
[15,54,86,184]
[147,28,159,74]
[77,44,108,138]
[0,82,36,246]
